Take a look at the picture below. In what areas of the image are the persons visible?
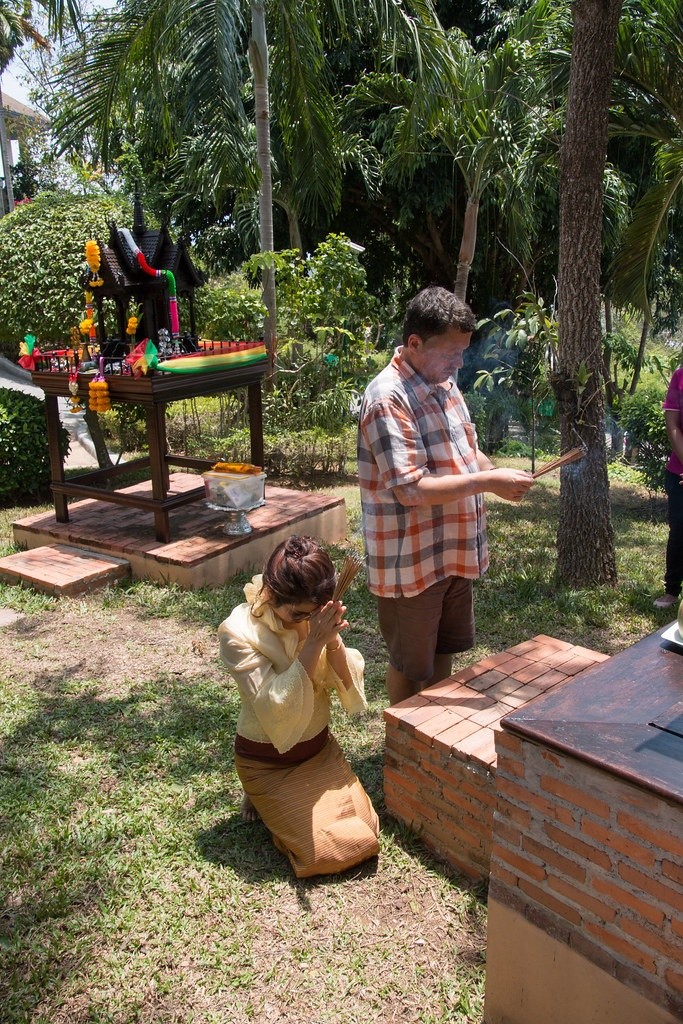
[218,536,379,877]
[654,366,683,608]
[358,287,535,704]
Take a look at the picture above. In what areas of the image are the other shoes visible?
[652,594,678,607]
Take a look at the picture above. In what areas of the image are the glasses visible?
[281,601,324,624]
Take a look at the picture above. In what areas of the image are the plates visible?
[661,622,683,648]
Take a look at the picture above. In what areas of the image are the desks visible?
[31,359,268,544]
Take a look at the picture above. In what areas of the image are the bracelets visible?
[326,639,342,651]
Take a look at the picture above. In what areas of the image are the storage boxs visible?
[203,469,266,509]
